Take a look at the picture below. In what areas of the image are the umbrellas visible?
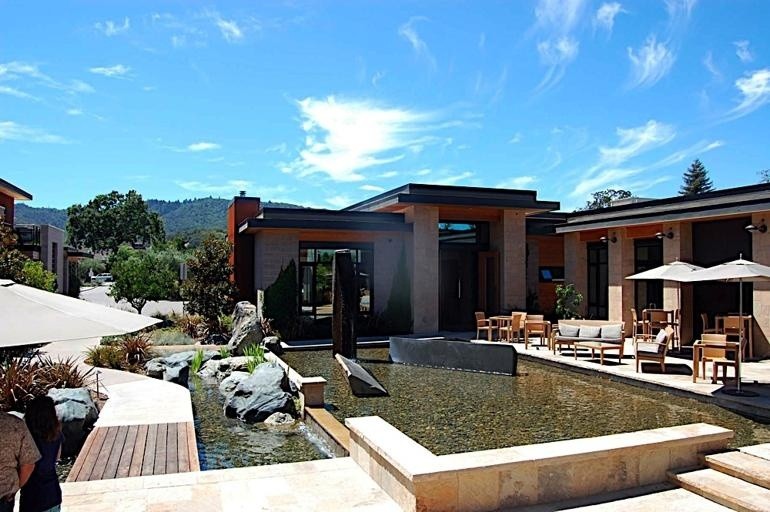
[0,275,168,355]
[679,254,770,392]
[622,257,708,347]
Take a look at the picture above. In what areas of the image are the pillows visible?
[559,322,623,338]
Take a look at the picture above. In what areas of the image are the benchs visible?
[634,327,675,373]
[551,319,626,357]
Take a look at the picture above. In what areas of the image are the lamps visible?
[600,237,617,243]
[655,232,674,239]
[745,224,768,234]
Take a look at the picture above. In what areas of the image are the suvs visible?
[91,273,114,282]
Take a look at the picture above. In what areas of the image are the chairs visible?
[475,312,551,349]
[693,313,752,384]
[631,308,680,349]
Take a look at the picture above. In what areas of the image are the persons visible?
[0,387,42,510]
[19,394,64,510]
